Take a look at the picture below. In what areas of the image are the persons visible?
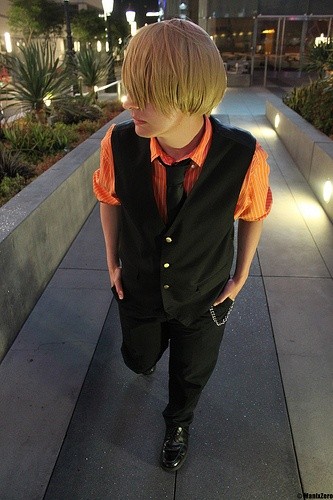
[91,19,272,473]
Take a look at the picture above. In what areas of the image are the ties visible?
[157,156,190,219]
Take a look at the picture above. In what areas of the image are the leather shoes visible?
[141,365,157,376]
[161,419,190,472]
